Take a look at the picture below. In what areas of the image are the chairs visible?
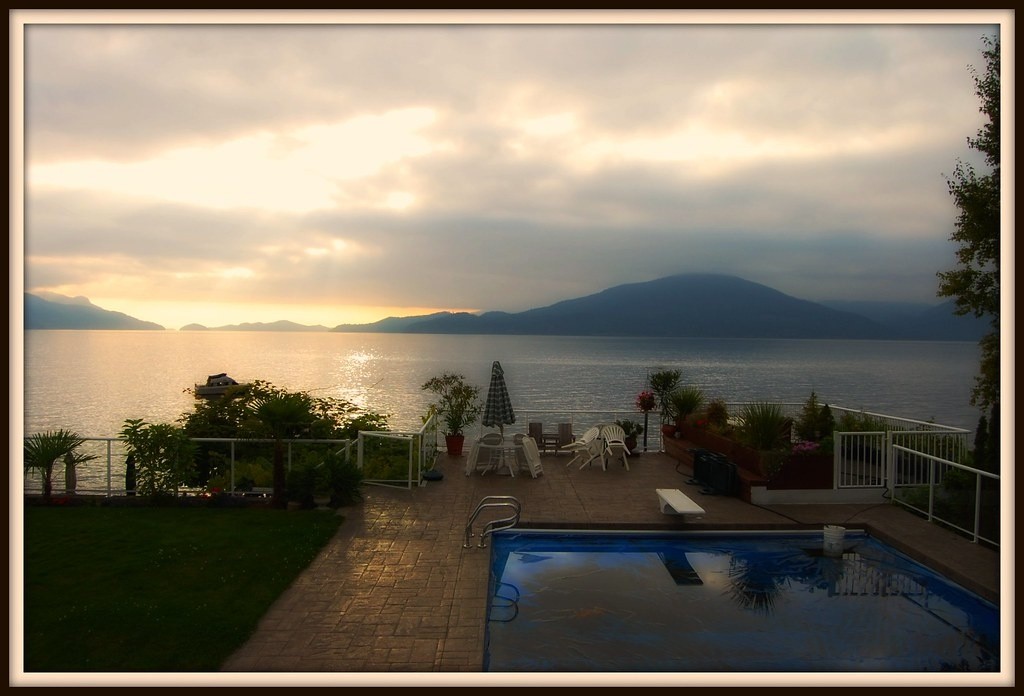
[466,422,631,478]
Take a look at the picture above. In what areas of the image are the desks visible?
[479,444,523,478]
[589,434,629,469]
[543,434,557,455]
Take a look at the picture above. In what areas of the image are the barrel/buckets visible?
[824,526,845,557]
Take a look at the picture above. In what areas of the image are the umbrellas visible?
[481,360,517,466]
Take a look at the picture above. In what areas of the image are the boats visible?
[193,374,253,397]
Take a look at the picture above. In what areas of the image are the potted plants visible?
[421,371,484,455]
[24,419,335,513]
[615,420,644,450]
